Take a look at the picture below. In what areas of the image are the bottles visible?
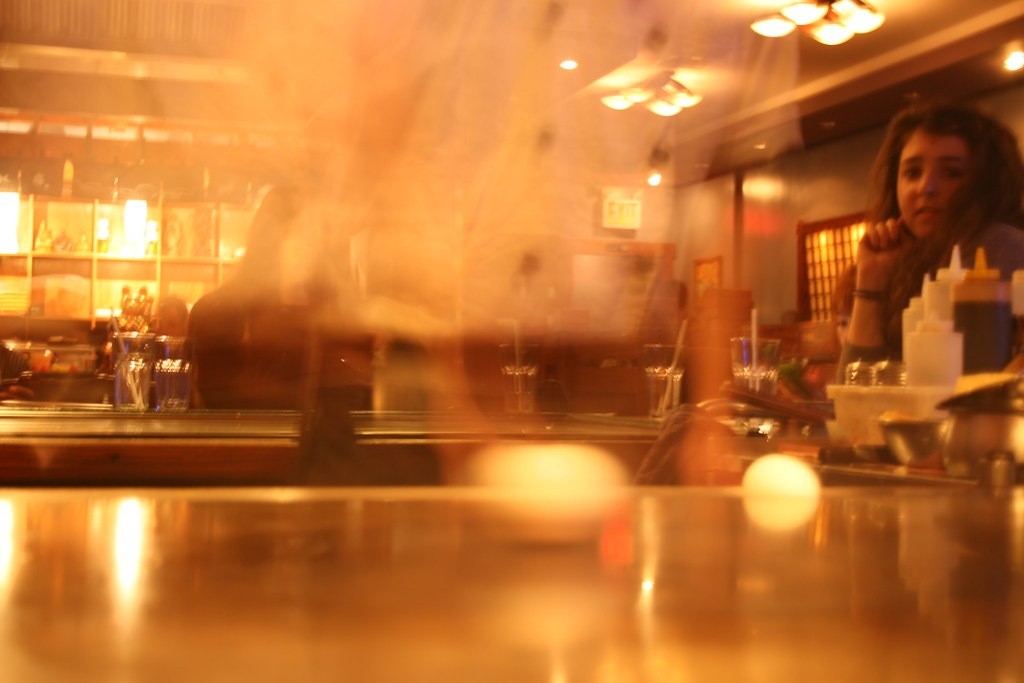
[898,243,1014,385]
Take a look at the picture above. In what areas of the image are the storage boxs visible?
[825,385,951,442]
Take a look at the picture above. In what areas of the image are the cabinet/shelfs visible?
[0,192,243,330]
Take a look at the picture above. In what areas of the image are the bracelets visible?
[852,288,891,300]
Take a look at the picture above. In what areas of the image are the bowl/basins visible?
[825,384,946,452]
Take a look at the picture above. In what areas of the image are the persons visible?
[830,102,1024,405]
[834,264,912,365]
[182,172,379,413]
[145,298,191,362]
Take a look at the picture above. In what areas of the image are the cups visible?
[642,343,685,422]
[111,331,155,412]
[154,335,193,414]
[728,335,781,437]
[498,342,540,412]
